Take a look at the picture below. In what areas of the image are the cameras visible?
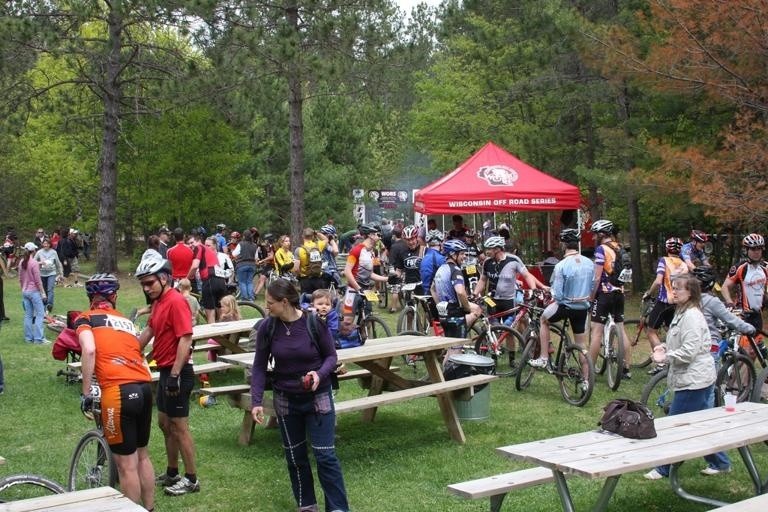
[300,376,314,390]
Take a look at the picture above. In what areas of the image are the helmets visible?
[230,231,241,238]
[133,258,172,281]
[84,273,121,295]
[216,224,227,230]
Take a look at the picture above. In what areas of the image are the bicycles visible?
[0,475,67,505]
[397,273,767,403]
[250,260,393,339]
[62,385,116,502]
[1,245,26,278]
[183,287,265,323]
[38,304,67,333]
[226,270,243,299]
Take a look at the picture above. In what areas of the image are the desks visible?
[0,485,151,512]
[136,317,263,345]
[494,401,768,512]
[216,335,473,448]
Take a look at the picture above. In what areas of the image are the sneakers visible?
[154,472,182,487]
[581,380,589,399]
[642,469,664,480]
[164,477,200,495]
[647,365,667,375]
[527,357,550,369]
[700,467,732,475]
[621,368,631,380]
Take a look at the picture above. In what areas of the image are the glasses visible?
[140,279,159,287]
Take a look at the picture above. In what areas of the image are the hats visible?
[25,242,38,251]
[158,228,173,234]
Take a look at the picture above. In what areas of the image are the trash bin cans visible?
[447,353,496,421]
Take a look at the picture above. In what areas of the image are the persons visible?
[130,213,429,362]
[250,277,350,510]
[641,272,733,481]
[678,228,711,272]
[721,233,768,394]
[0,228,93,397]
[642,237,691,375]
[694,266,758,408]
[134,257,201,497]
[74,270,155,512]
[415,214,632,392]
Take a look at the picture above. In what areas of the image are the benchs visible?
[200,364,400,397]
[334,374,497,414]
[447,466,567,501]
[67,337,256,370]
[151,361,232,382]
[703,492,765,512]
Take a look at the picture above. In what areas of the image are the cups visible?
[724,395,737,412]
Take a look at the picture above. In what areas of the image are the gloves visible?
[164,374,181,397]
[79,392,95,420]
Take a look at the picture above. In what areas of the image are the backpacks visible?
[298,241,323,278]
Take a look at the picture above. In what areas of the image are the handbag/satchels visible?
[597,399,657,440]
